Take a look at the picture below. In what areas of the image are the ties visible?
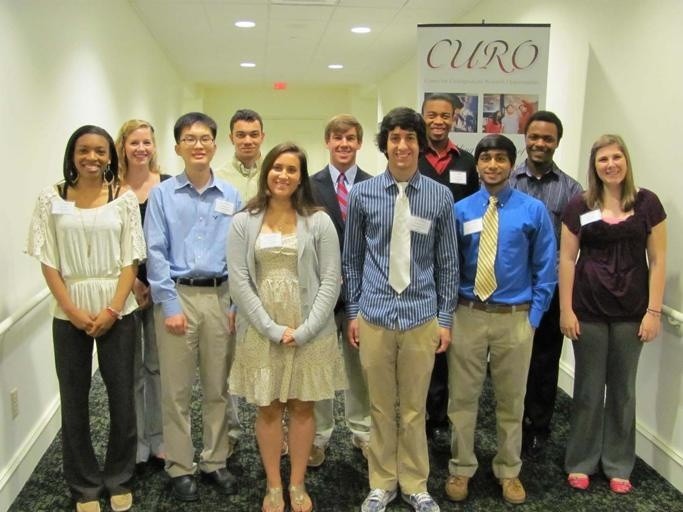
[388,182,411,294]
[474,194,498,302]
[336,174,350,224]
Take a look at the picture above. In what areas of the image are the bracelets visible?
[106,306,123,320]
[646,307,663,316]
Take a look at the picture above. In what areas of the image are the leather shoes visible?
[525,435,544,461]
[172,475,198,501]
[228,458,241,471]
[201,469,238,494]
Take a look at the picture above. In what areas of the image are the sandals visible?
[261,485,285,512]
[288,485,313,512]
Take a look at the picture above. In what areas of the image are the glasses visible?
[177,134,216,146]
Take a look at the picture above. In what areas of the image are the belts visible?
[458,299,531,315]
[169,276,229,286]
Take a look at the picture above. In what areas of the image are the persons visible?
[518,100,535,134]
[416,94,478,441]
[486,110,502,132]
[444,133,558,504]
[226,144,343,512]
[562,135,666,494]
[506,111,581,461]
[342,107,458,512]
[145,111,244,501]
[25,124,147,511]
[307,114,378,469]
[115,121,172,481]
[214,111,289,469]
[501,103,520,133]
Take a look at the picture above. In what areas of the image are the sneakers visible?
[306,441,329,467]
[445,473,468,500]
[611,478,631,493]
[568,474,589,491]
[401,489,440,512]
[76,497,101,512]
[352,433,370,460]
[433,430,449,447]
[280,426,290,456]
[361,487,397,512]
[109,488,132,511]
[502,477,526,504]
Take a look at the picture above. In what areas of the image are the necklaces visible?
[69,186,106,259]
[269,204,293,232]
